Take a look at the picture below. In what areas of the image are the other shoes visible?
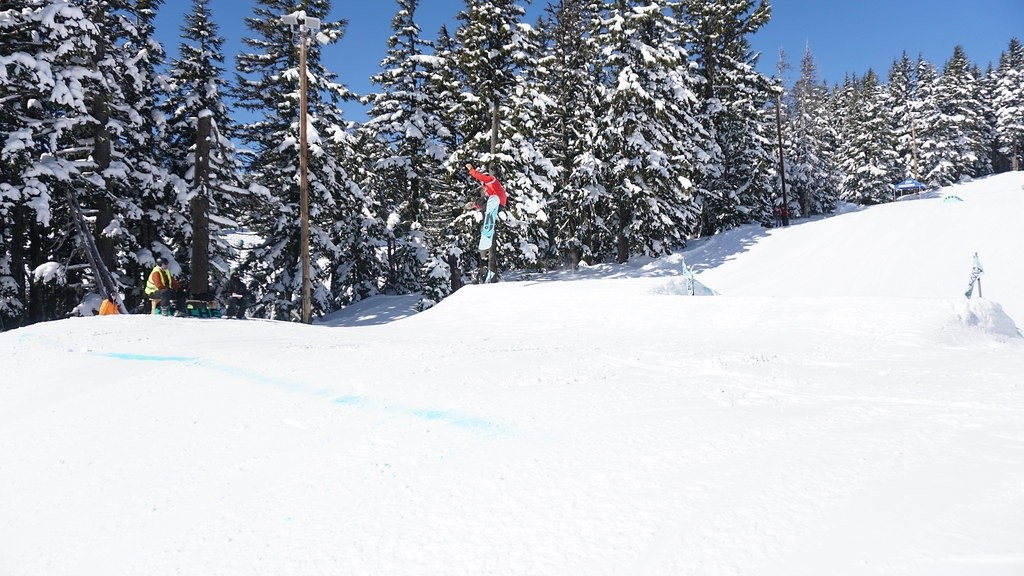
[160,306,169,317]
[173,310,185,317]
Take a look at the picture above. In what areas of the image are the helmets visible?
[156,257,170,263]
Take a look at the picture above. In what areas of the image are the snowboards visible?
[477,194,500,252]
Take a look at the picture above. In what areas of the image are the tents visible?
[894,178,925,201]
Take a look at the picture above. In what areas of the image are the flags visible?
[964,257,982,298]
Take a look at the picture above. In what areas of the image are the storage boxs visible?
[185,300,222,318]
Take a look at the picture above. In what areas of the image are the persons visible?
[145,258,186,317]
[761,205,789,228]
[99,290,118,315]
[466,163,506,212]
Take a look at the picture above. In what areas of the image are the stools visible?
[150,299,162,314]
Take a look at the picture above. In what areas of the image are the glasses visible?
[164,262,169,265]
[111,292,117,296]
